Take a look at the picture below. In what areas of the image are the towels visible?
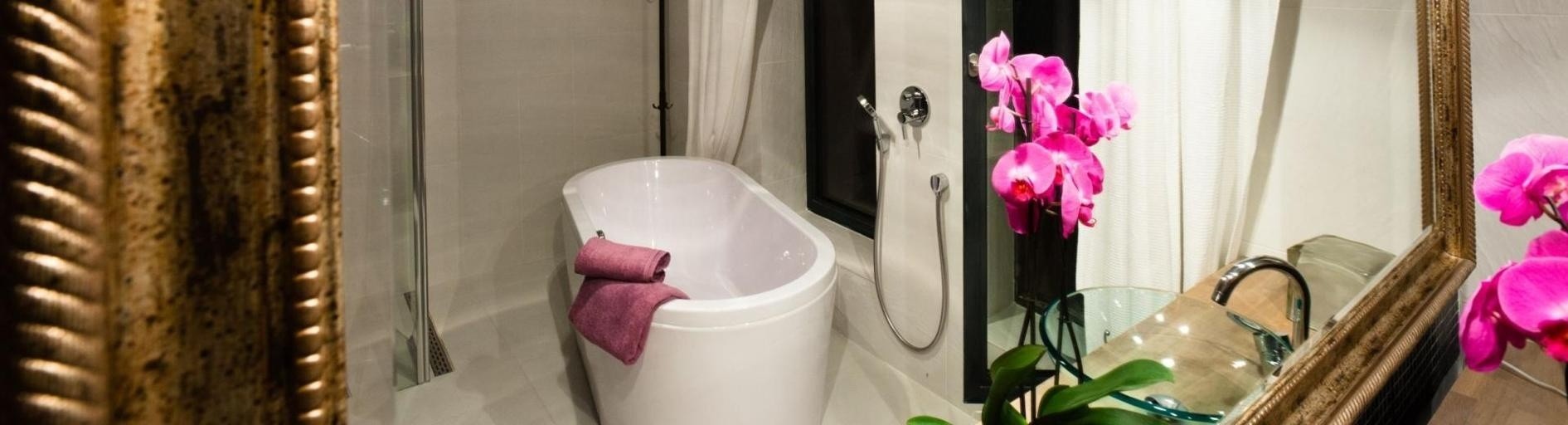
[567,235,691,365]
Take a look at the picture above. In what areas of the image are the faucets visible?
[1214,254,1311,350]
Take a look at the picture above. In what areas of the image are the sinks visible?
[1041,287,1290,424]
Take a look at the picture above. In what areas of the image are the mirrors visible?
[5,3,1476,425]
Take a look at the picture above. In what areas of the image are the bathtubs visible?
[560,155,838,425]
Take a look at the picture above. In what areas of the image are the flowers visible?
[896,24,1198,424]
[1459,131,1567,376]
